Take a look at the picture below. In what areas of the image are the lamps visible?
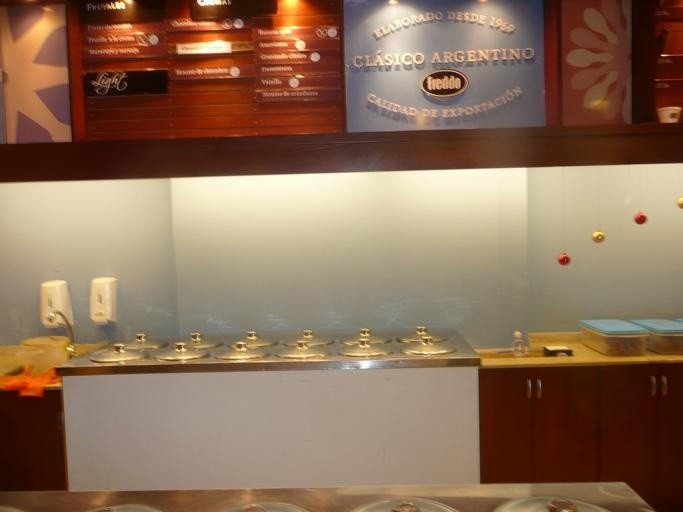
[634,164,647,224]
[558,167,570,266]
[678,163,683,209]
[591,166,604,242]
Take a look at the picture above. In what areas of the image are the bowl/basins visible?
[657,106,681,122]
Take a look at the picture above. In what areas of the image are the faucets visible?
[47,310,77,360]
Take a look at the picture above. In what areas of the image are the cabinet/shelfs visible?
[475,332,683,483]
[0,342,109,491]
